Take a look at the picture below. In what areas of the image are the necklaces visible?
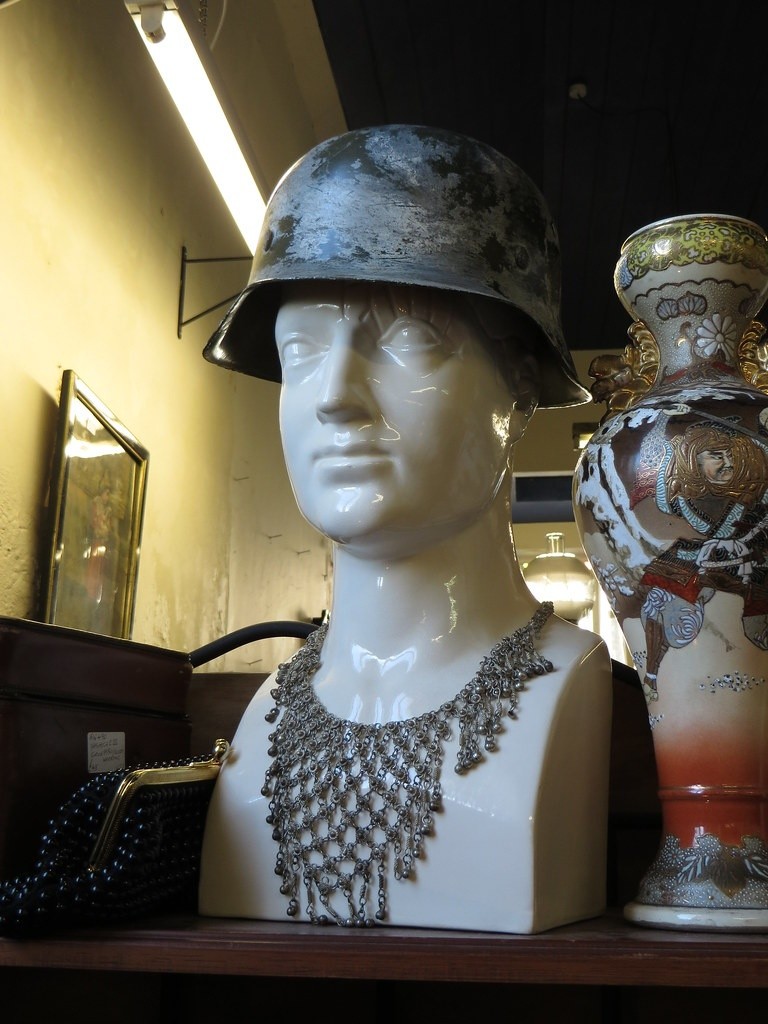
[260,597,558,938]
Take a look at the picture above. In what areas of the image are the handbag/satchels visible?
[0,737,237,942]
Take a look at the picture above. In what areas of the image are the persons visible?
[198,123,611,938]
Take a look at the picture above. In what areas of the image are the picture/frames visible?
[38,370,150,639]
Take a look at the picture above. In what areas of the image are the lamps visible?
[569,83,669,128]
[124,0,267,257]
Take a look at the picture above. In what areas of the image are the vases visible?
[571,214,768,932]
